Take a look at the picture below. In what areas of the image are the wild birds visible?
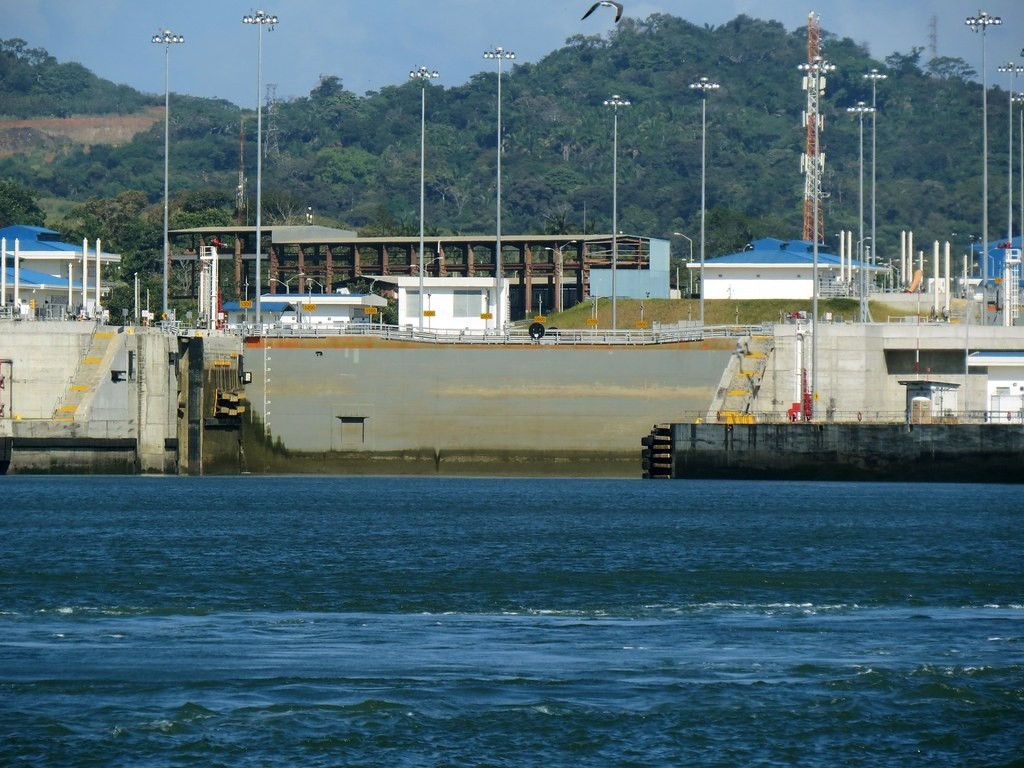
[580,1,624,23]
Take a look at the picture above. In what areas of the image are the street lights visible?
[688,76,721,330]
[480,47,516,336]
[150,28,186,329]
[410,257,444,276]
[408,65,439,336]
[1011,91,1023,281]
[603,93,630,336]
[834,230,997,294]
[863,67,887,265]
[307,278,324,294]
[241,8,278,327]
[965,10,1002,324]
[370,279,379,293]
[545,240,578,314]
[674,233,694,293]
[848,101,877,323]
[270,273,304,294]
[998,62,1023,248]
